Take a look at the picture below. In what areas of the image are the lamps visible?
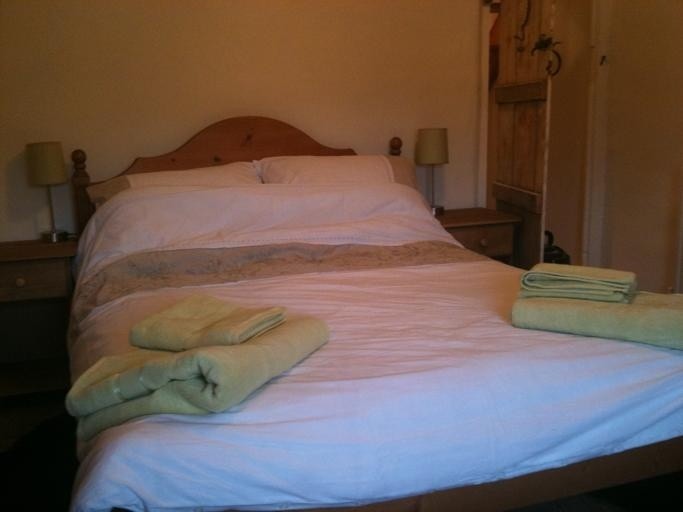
[413,127,451,217]
[22,140,70,245]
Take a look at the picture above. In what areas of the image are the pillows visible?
[252,150,418,189]
[87,161,266,211]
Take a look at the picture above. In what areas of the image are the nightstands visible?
[430,206,518,265]
[1,240,72,401]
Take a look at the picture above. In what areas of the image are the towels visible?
[509,286,682,357]
[119,288,287,349]
[65,312,329,439]
[516,261,635,299]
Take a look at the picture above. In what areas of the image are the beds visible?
[67,117,681,511]
